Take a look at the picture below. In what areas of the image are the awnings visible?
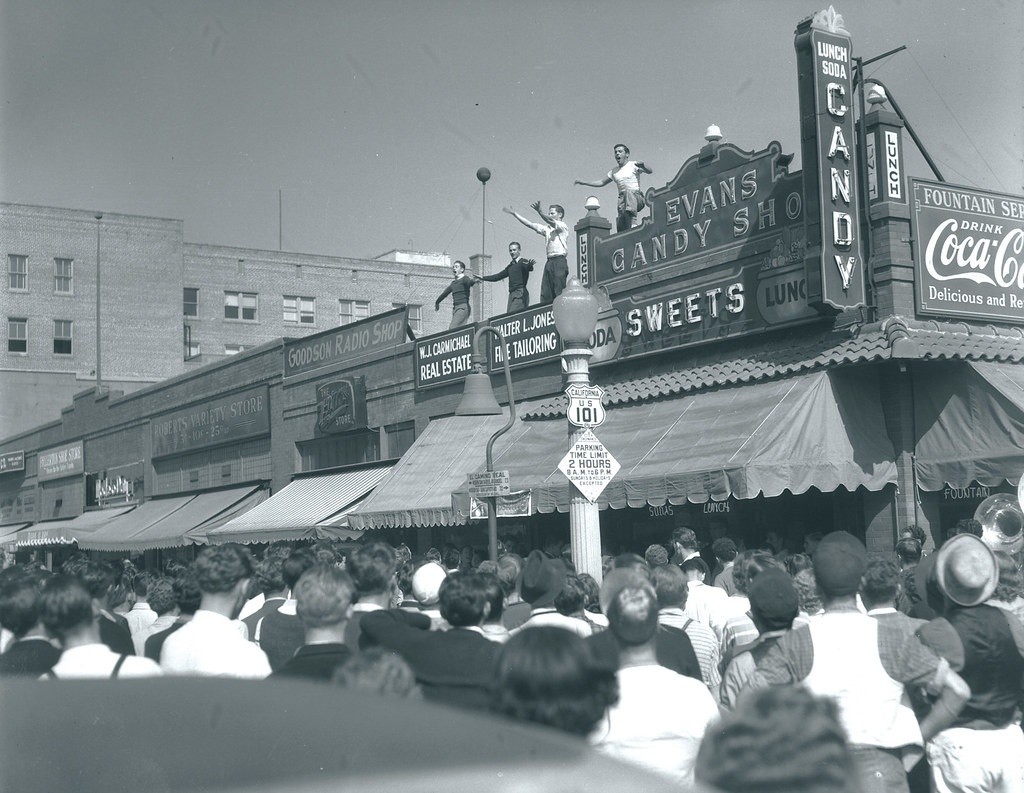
[2,359,1024,555]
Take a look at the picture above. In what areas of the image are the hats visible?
[516,549,566,605]
[599,567,658,643]
[748,567,799,627]
[412,563,447,604]
[811,531,868,591]
[936,533,999,606]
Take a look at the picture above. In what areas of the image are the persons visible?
[574,143,653,233]
[502,201,569,306]
[2,515,1024,791]
[473,240,536,315]
[434,260,483,331]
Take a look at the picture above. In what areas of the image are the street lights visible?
[551,275,604,586]
[93,210,105,386]
[476,167,491,323]
[453,323,518,562]
